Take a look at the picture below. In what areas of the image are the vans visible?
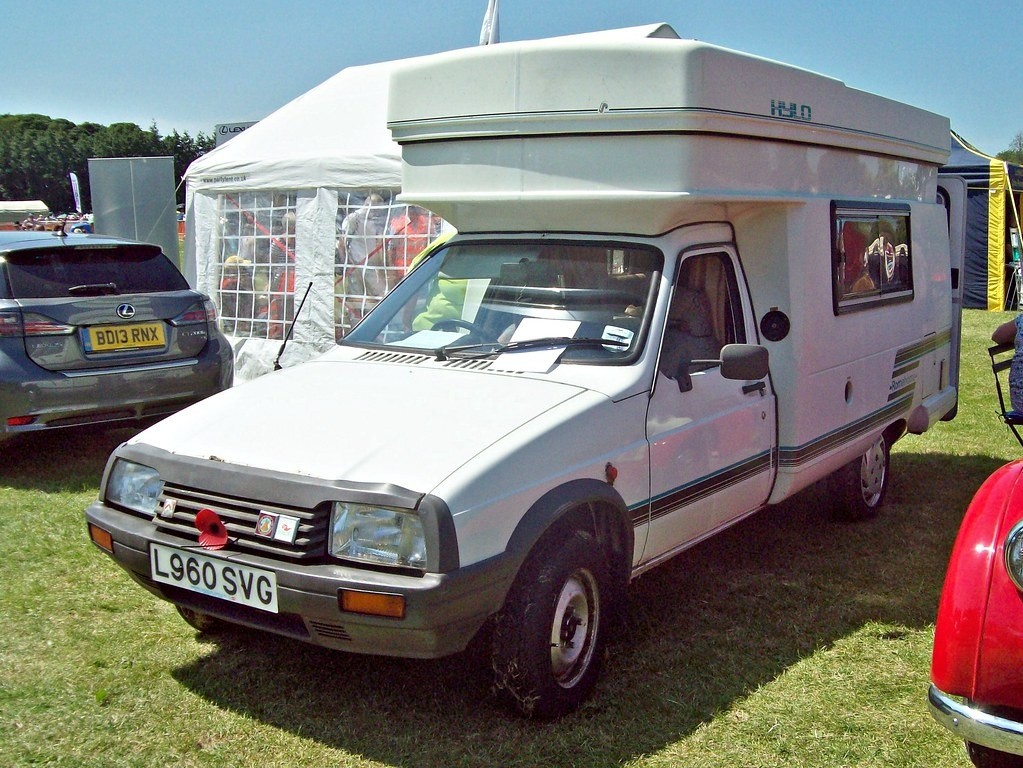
[88,25,970,726]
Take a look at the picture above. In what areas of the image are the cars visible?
[70,214,94,235]
[0,229,236,455]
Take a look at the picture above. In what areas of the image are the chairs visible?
[601,272,714,390]
[477,261,551,351]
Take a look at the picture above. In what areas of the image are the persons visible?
[991,311,1023,414]
[36,215,45,222]
[22,215,39,230]
[1006,244,1017,303]
[45,212,56,221]
[220,190,442,340]
